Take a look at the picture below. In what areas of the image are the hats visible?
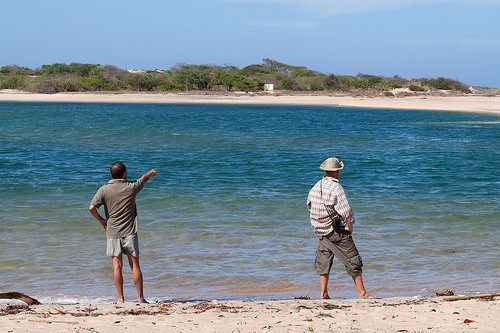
[319,157,344,171]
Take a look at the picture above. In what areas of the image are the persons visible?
[89,161,157,304]
[307,156,375,299]
